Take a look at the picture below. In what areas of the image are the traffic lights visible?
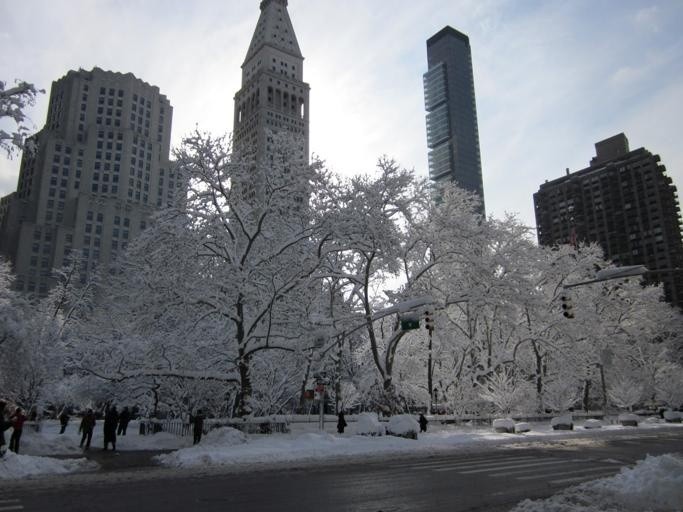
[423,308,434,331]
[304,389,315,399]
[560,290,575,319]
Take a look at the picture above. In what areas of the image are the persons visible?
[337,411,347,433]
[193,410,203,446]
[0,402,132,458]
[419,414,428,432]
